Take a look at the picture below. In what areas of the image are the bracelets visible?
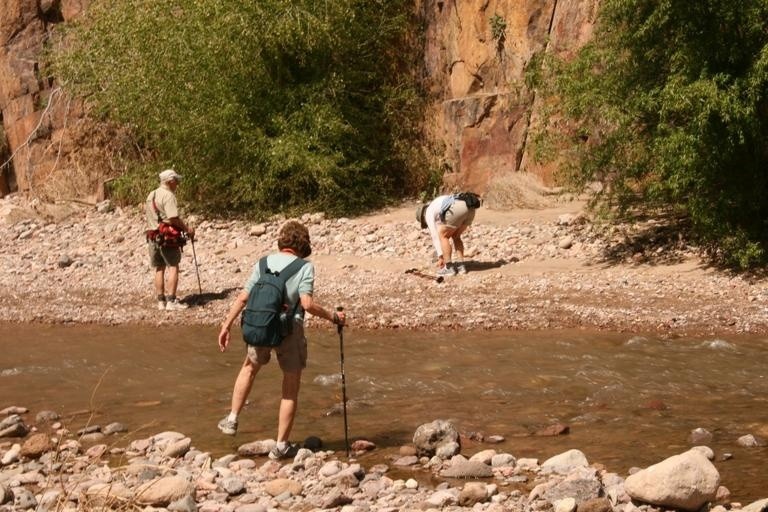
[332,311,342,324]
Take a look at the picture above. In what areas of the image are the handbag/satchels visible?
[458,192,482,209]
[158,223,186,253]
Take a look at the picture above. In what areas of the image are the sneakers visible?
[165,299,187,310]
[267,442,299,462]
[436,266,455,276]
[217,417,237,436]
[158,300,166,310]
[456,264,466,275]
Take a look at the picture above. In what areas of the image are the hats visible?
[415,204,429,228]
[158,169,182,184]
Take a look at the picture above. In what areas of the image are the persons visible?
[215,222,346,459]
[144,168,197,312]
[413,193,475,276]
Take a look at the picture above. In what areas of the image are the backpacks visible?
[240,255,308,346]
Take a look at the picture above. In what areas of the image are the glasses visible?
[174,181,180,185]
